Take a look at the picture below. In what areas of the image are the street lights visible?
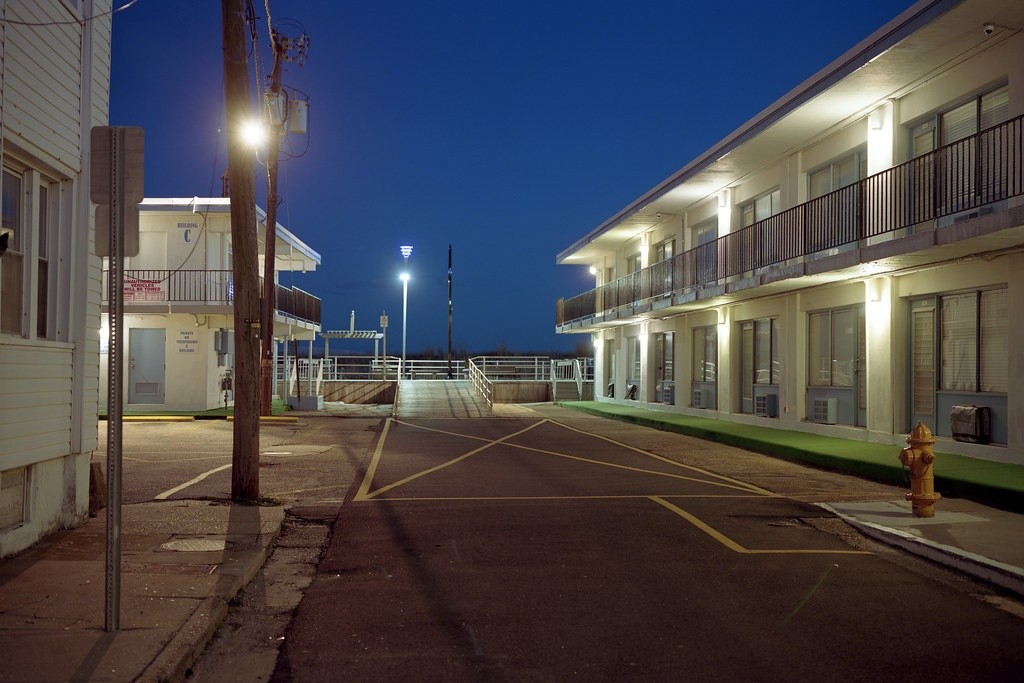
[398,244,413,378]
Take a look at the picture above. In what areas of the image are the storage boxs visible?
[694,389,707,409]
[950,405,991,444]
[813,397,837,425]
[663,388,674,405]
[755,393,777,418]
